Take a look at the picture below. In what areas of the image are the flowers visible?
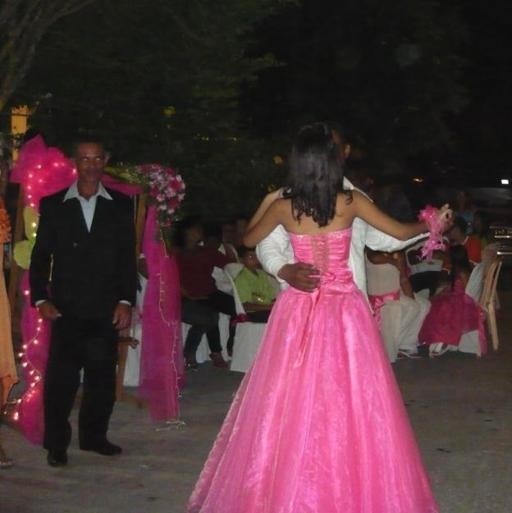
[105,162,187,256]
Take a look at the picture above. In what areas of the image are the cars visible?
[451,184,512,267]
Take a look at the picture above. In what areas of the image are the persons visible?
[189,119,453,513]
[0,121,490,469]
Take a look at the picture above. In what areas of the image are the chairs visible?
[181,242,269,372]
[123,273,149,388]
[364,252,503,363]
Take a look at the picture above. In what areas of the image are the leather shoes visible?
[46,449,68,466]
[94,441,122,457]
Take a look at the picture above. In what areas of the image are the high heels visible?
[209,351,228,366]
[184,354,197,367]
